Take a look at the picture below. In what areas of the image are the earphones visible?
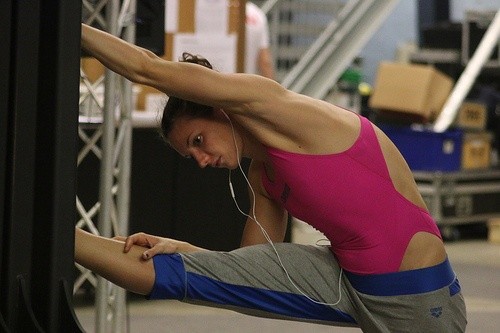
[220,108,229,119]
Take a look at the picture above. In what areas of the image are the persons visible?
[75,23,468,333]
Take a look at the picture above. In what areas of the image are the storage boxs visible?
[459,131,493,171]
[366,60,454,121]
[455,100,487,131]
[377,123,461,173]
